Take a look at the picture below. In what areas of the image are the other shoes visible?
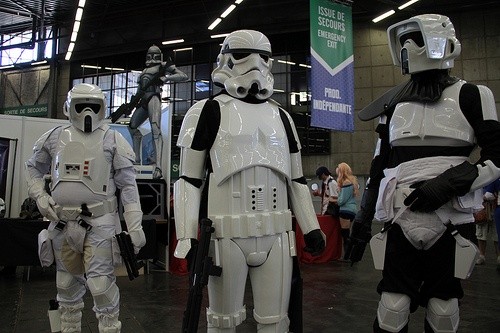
[475,254,487,265]
[336,257,352,266]
[496,255,500,266]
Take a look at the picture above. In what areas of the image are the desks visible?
[0,216,157,282]
[296,214,343,265]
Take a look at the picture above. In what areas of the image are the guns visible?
[107,51,175,123]
[115,232,146,282]
[181,218,223,333]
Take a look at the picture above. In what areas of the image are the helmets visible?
[386,14,461,75]
[62,82,108,134]
[144,44,164,66]
[211,30,275,102]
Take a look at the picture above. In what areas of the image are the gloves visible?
[46,198,59,224]
[185,240,212,272]
[303,229,327,257]
[342,219,373,262]
[123,210,146,255]
[400,160,480,213]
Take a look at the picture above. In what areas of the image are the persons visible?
[24,84,147,333]
[356,16,500,333]
[330,161,358,228]
[314,167,338,213]
[127,45,189,180]
[174,31,324,333]
[475,180,500,266]
